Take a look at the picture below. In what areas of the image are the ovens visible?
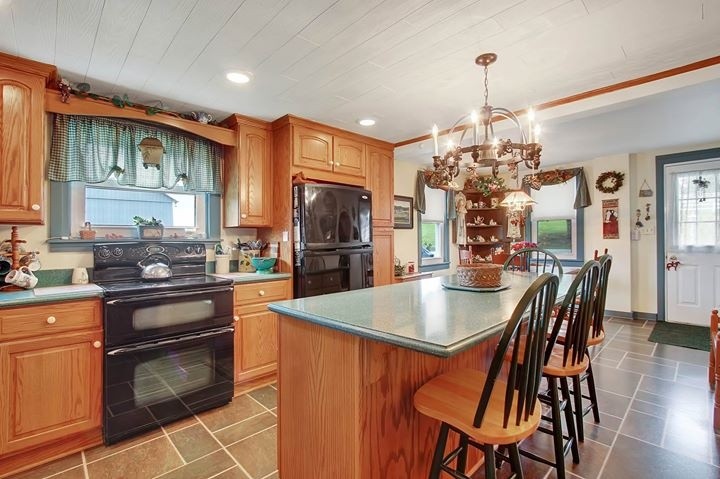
[104,288,235,446]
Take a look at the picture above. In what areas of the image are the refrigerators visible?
[293,183,375,299]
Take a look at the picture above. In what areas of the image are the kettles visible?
[136,252,172,281]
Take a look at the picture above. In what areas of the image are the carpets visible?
[648,322,720,351]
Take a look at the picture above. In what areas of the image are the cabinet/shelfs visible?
[0,297,104,479]
[0,52,56,225]
[219,112,432,397]
[458,189,527,267]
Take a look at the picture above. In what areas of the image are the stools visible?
[413,248,613,479]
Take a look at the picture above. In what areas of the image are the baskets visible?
[456,264,503,286]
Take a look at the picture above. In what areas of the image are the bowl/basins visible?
[215,254,238,274]
[248,257,276,271]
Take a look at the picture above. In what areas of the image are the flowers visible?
[513,242,537,270]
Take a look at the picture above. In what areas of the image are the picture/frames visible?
[393,195,414,229]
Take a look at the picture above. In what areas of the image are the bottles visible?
[408,262,414,274]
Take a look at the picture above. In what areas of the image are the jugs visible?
[393,258,406,275]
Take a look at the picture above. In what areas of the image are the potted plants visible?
[134,216,163,241]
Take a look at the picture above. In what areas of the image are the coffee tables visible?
[708,310,720,434]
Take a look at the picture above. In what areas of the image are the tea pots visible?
[474,216,484,225]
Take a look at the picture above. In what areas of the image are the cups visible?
[460,250,469,264]
[0,261,11,277]
[465,198,499,208]
[238,247,255,273]
[71,267,89,285]
[5,266,38,289]
[490,221,496,226]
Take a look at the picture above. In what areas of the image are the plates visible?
[440,273,513,290]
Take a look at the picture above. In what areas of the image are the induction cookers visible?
[94,242,233,297]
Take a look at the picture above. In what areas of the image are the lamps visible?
[430,51,543,187]
[499,190,539,256]
